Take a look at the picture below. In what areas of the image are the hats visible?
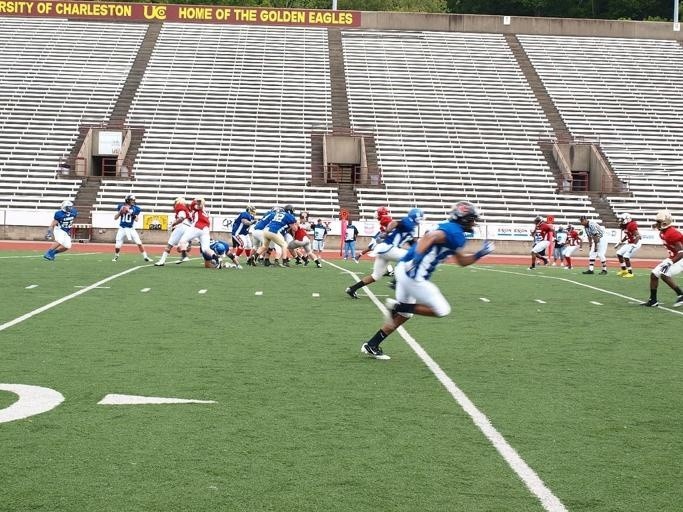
[579,216,587,219]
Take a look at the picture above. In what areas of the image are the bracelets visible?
[475,252,481,259]
[412,253,423,261]
[667,260,673,266]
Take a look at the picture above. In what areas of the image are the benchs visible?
[0,15,683,236]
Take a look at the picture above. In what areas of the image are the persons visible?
[360,201,495,361]
[640,211,683,307]
[527,218,582,270]
[154,196,393,276]
[580,216,608,275]
[112,194,154,262]
[613,213,642,278]
[44,201,77,260]
[346,209,429,299]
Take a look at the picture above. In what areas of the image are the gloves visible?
[660,261,671,273]
[46,234,51,240]
[474,239,495,259]
[404,258,419,280]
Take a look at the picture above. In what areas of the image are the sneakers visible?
[43,254,51,259]
[360,343,391,360]
[175,256,190,264]
[598,270,607,275]
[552,262,573,270]
[113,256,119,262]
[582,269,593,274]
[616,270,627,276]
[672,292,682,308]
[155,261,165,266]
[295,257,322,268]
[214,257,224,269]
[245,256,292,268]
[345,287,360,299]
[386,299,401,319]
[528,259,550,271]
[622,272,633,278]
[640,297,658,307]
[144,256,153,262]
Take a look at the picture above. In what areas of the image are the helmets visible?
[125,194,136,207]
[61,200,74,212]
[653,209,673,231]
[175,196,187,205]
[615,213,632,226]
[448,202,478,233]
[213,243,224,255]
[374,207,423,231]
[246,204,294,216]
[534,216,544,227]
[566,224,574,232]
[194,197,205,207]
